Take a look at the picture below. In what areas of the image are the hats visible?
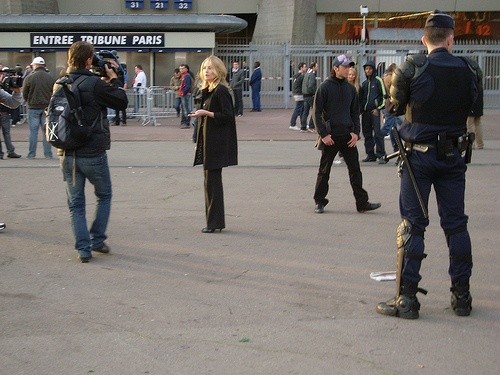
[30,57,45,65]
[333,54,355,67]
[425,9,455,29]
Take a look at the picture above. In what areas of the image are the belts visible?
[413,143,436,153]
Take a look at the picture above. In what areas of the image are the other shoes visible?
[110,121,117,126]
[92,244,108,253]
[0,224,6,231]
[308,128,318,134]
[306,125,309,129]
[362,157,376,162]
[357,203,381,212]
[314,203,324,212]
[289,125,300,130]
[8,153,21,158]
[80,257,90,263]
[250,109,258,112]
[181,125,190,129]
[379,160,385,164]
[300,130,307,132]
[0,155,3,159]
[121,122,126,126]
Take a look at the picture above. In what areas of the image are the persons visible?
[248,61,262,112]
[312,54,381,213]
[51,41,129,263]
[466,68,484,150]
[230,62,244,118]
[176,64,191,129]
[242,62,249,97]
[111,64,147,127]
[0,221,6,231]
[290,60,296,91]
[374,63,404,153]
[186,64,195,82]
[298,62,318,133]
[334,68,363,164]
[168,68,182,118]
[361,60,387,164]
[0,56,66,160]
[376,9,479,319]
[187,56,238,233]
[289,62,309,131]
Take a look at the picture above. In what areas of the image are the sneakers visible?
[453,291,472,315]
[377,295,419,318]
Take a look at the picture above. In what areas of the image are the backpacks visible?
[46,75,100,151]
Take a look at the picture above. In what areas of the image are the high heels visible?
[202,228,222,233]
